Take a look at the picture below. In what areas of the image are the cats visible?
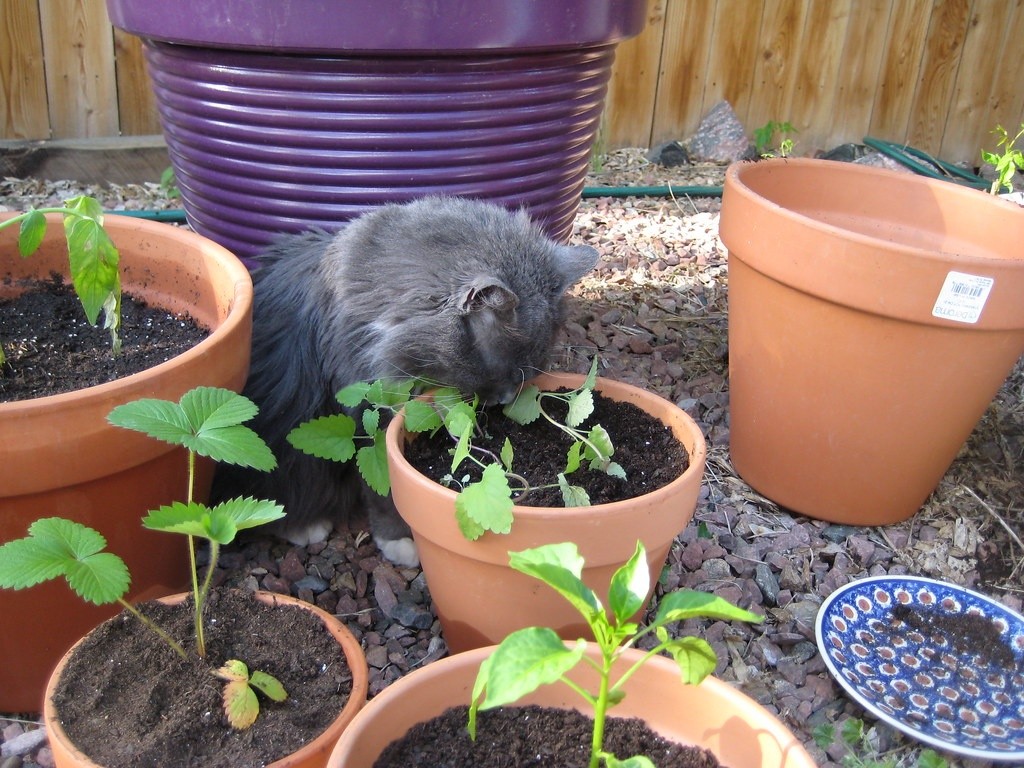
[208,193,603,548]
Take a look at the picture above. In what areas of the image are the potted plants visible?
[0,194,817,768]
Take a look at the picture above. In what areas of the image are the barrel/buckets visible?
[720,154,1024,523]
[104,0,649,285]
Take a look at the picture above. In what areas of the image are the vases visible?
[718,156,1024,528]
[107,0,648,267]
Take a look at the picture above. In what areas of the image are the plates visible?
[814,575,1024,763]
